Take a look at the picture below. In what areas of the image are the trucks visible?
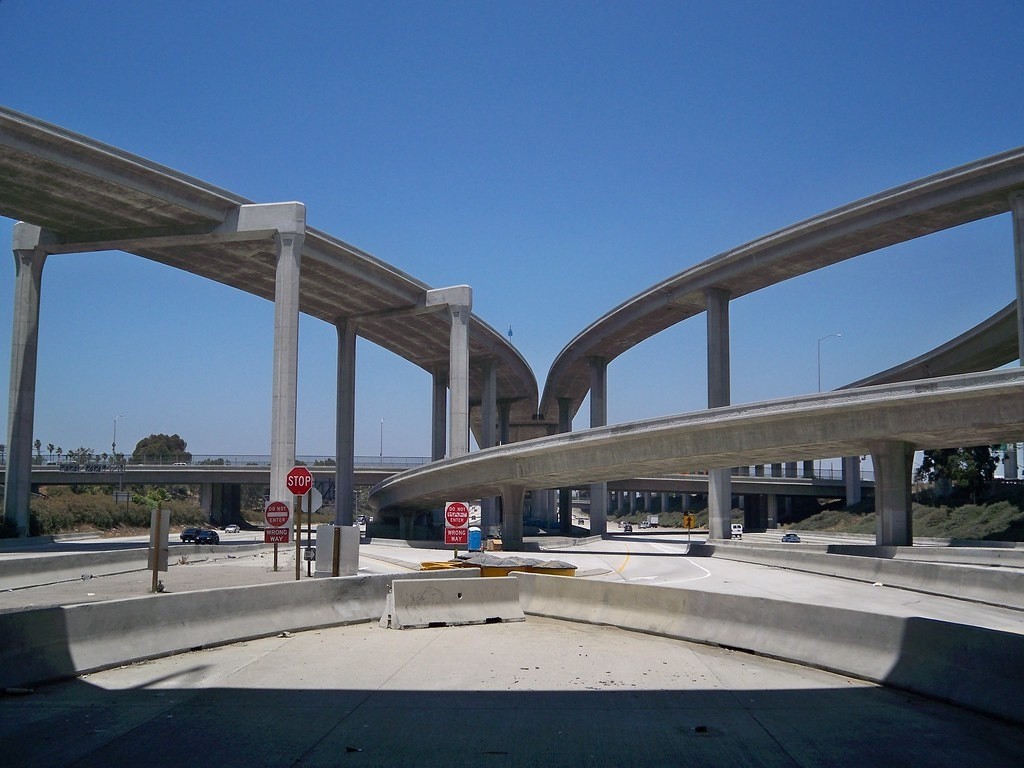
[647,514,658,529]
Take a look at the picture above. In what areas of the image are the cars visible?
[578,519,584,525]
[180,528,203,543]
[618,521,633,533]
[225,524,240,533]
[195,531,220,545]
[638,520,651,529]
[782,533,800,543]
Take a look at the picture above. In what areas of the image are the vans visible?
[172,463,187,465]
[731,523,743,537]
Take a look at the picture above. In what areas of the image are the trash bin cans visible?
[468,527,481,551]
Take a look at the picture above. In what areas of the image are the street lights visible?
[818,333,843,392]
[380,416,385,467]
[113,414,124,443]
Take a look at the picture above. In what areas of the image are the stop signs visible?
[265,502,289,528]
[287,466,313,496]
